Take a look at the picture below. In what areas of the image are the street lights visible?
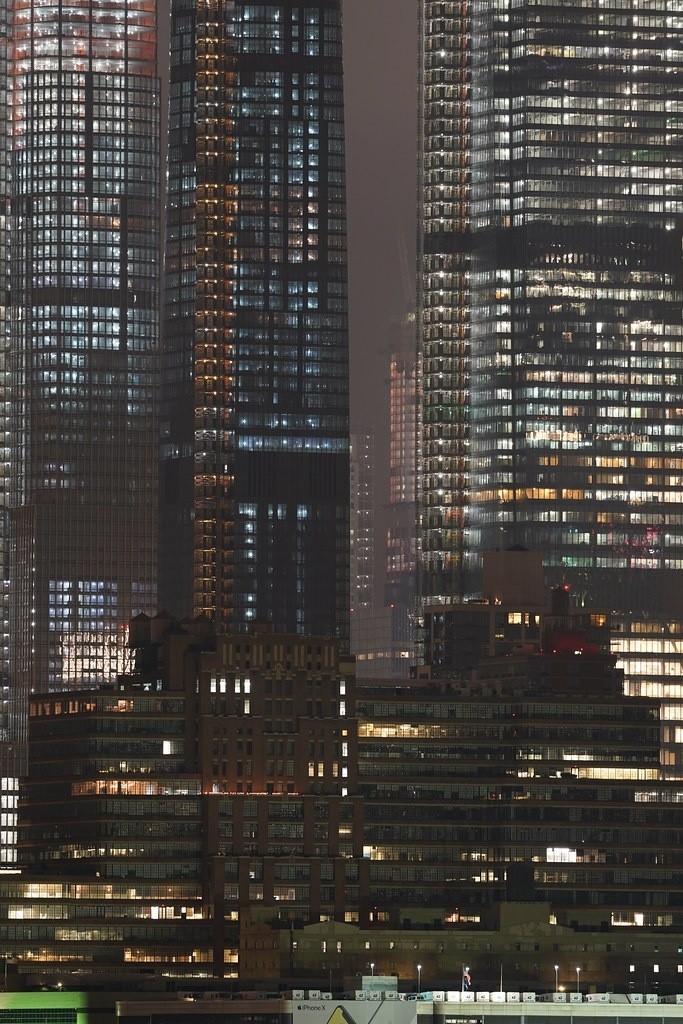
[554,966,559,992]
[576,967,581,993]
[323,964,332,992]
[462,964,469,991]
[371,963,374,991]
[417,965,421,991]
